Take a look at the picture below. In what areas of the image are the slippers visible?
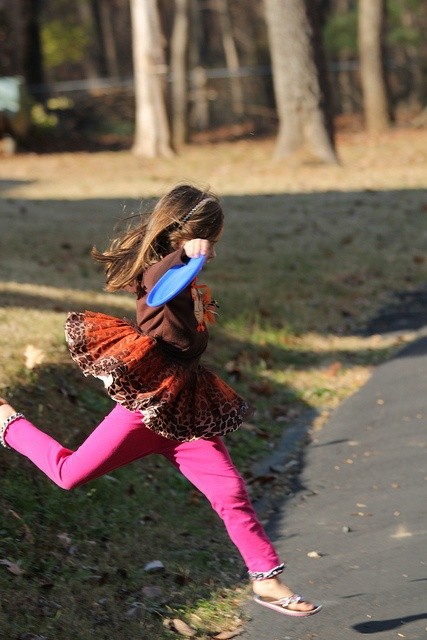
[254,592,322,617]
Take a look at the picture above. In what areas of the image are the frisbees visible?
[144,255,205,307]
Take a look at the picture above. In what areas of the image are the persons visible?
[0,187,323,617]
